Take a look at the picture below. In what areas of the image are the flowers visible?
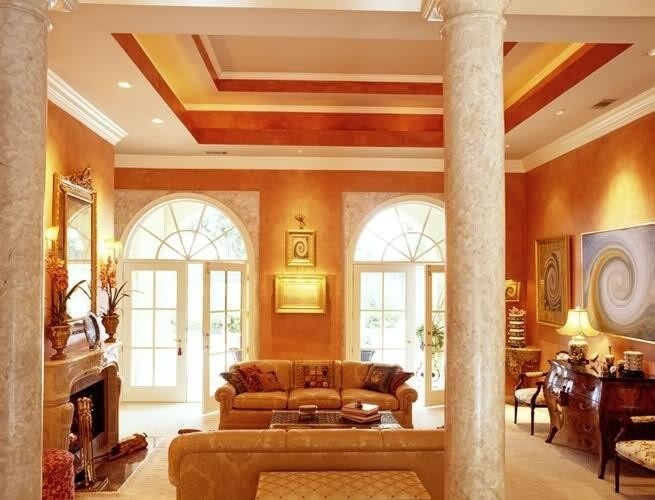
[42,258,86,326]
[91,254,144,317]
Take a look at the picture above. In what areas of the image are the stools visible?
[42,449,76,500]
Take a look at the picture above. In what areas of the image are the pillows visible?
[219,364,283,393]
[303,364,329,389]
[361,365,415,395]
[178,429,201,434]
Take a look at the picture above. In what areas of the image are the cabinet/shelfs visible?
[543,358,655,479]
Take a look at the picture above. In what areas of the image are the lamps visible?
[554,305,602,363]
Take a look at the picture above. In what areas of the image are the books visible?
[340,402,380,423]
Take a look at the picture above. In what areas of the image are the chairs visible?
[514,350,571,435]
[612,415,655,493]
[361,350,376,363]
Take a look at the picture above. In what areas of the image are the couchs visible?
[214,359,418,430]
[167,429,445,500]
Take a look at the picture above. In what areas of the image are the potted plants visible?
[416,320,446,351]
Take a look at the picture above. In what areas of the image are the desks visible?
[505,346,542,407]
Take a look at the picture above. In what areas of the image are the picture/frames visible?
[285,229,316,268]
[274,271,327,314]
[534,234,572,329]
[580,222,655,345]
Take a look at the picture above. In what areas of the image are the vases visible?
[101,314,120,343]
[48,326,71,360]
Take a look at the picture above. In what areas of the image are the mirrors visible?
[52,165,98,336]
